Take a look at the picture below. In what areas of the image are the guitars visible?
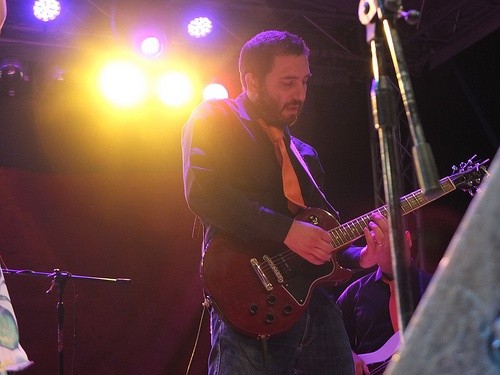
[202,152,498,344]
[356,327,402,375]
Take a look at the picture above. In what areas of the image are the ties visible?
[257,117,307,216]
[383,276,400,333]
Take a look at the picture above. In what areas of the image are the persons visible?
[176,29,394,375]
[329,217,436,374]
[1,0,38,375]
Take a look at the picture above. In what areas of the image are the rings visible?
[376,239,385,247]
